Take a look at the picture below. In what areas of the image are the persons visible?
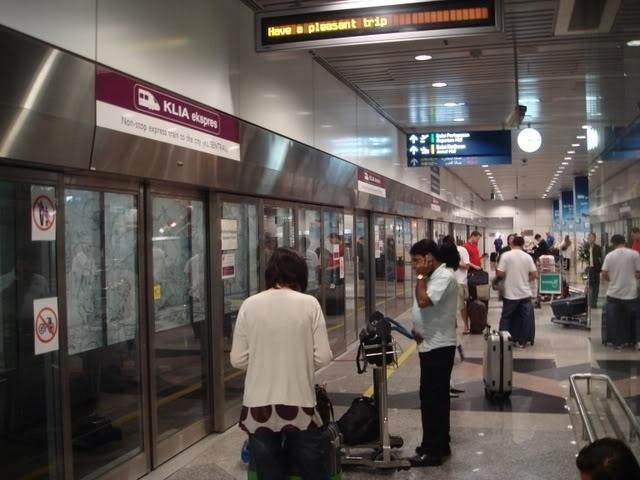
[575,437,639,479]
[401,239,460,467]
[586,232,603,309]
[183,232,396,318]
[599,233,640,352]
[628,226,640,251]
[228,246,336,480]
[440,230,575,346]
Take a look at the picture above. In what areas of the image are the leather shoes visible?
[450,394,459,398]
[408,455,443,467]
[415,446,451,457]
[451,387,464,393]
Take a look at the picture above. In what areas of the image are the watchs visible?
[416,274,426,280]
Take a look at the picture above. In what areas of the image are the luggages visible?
[600,304,612,346]
[483,323,514,401]
[511,301,535,346]
[327,423,344,480]
[467,299,487,334]
[549,295,586,320]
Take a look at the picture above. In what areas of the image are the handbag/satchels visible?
[338,395,379,446]
[315,383,334,432]
[356,311,417,374]
[469,269,488,287]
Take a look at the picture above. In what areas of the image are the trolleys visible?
[549,273,594,331]
[337,312,412,471]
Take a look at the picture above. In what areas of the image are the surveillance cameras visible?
[505,105,527,129]
